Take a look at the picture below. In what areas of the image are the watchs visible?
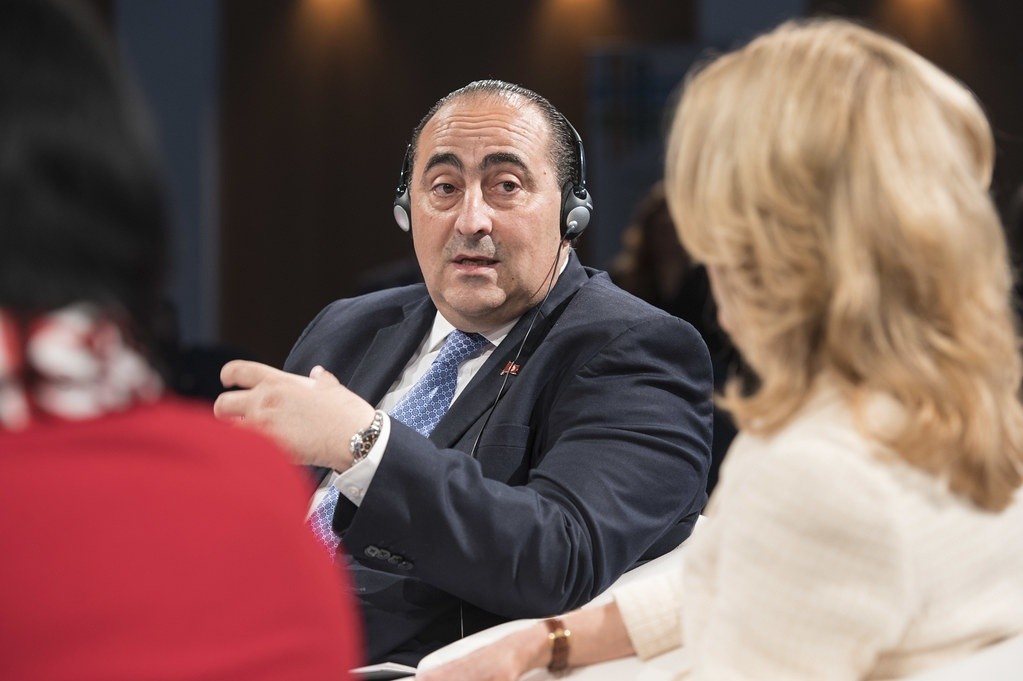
[351,412,383,466]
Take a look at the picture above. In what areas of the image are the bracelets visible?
[546,619,570,672]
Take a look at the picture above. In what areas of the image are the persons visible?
[212,80,715,666]
[414,20,1023,681]
[1,0,365,681]
[613,180,715,348]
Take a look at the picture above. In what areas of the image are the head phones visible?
[391,107,594,240]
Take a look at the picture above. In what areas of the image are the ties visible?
[306,328,491,561]
